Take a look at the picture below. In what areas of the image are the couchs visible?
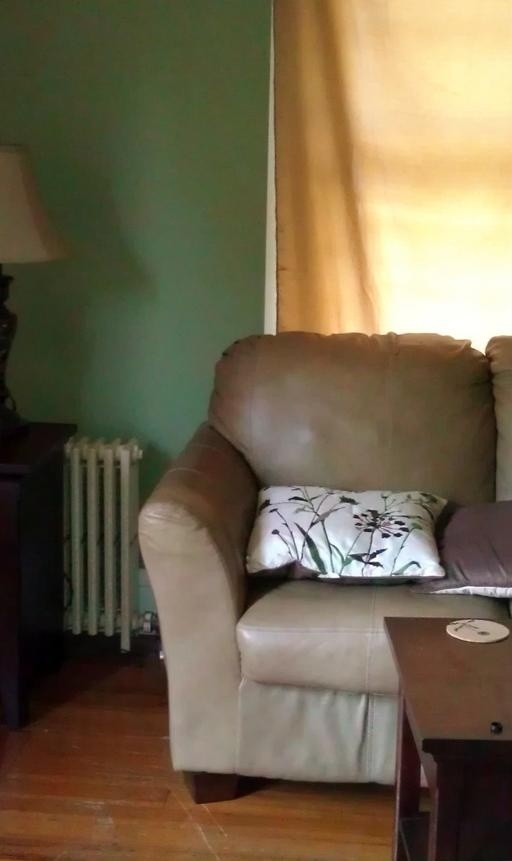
[138,330,512,804]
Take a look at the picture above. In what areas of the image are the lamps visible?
[0,144,65,440]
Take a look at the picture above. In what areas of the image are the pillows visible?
[413,500,512,599]
[244,483,449,587]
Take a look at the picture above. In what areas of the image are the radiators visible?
[63,430,157,654]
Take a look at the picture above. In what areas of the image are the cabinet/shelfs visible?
[0,423,78,730]
